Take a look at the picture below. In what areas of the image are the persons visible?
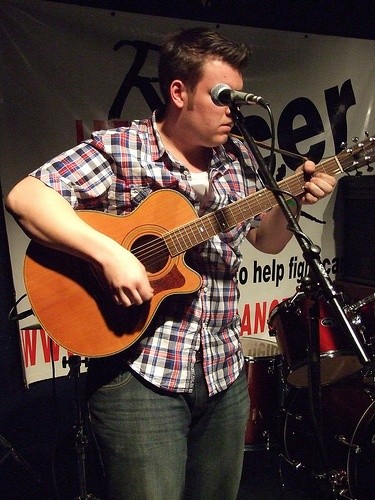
[5,26,336,499]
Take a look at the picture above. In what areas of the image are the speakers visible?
[341,176,375,287]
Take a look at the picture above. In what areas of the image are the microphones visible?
[211,83,269,109]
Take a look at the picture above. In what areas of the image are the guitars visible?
[22,134,375,358]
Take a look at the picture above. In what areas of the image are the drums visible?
[267,285,363,386]
[237,337,284,452]
[284,372,375,499]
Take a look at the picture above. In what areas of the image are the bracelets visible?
[288,199,296,206]
